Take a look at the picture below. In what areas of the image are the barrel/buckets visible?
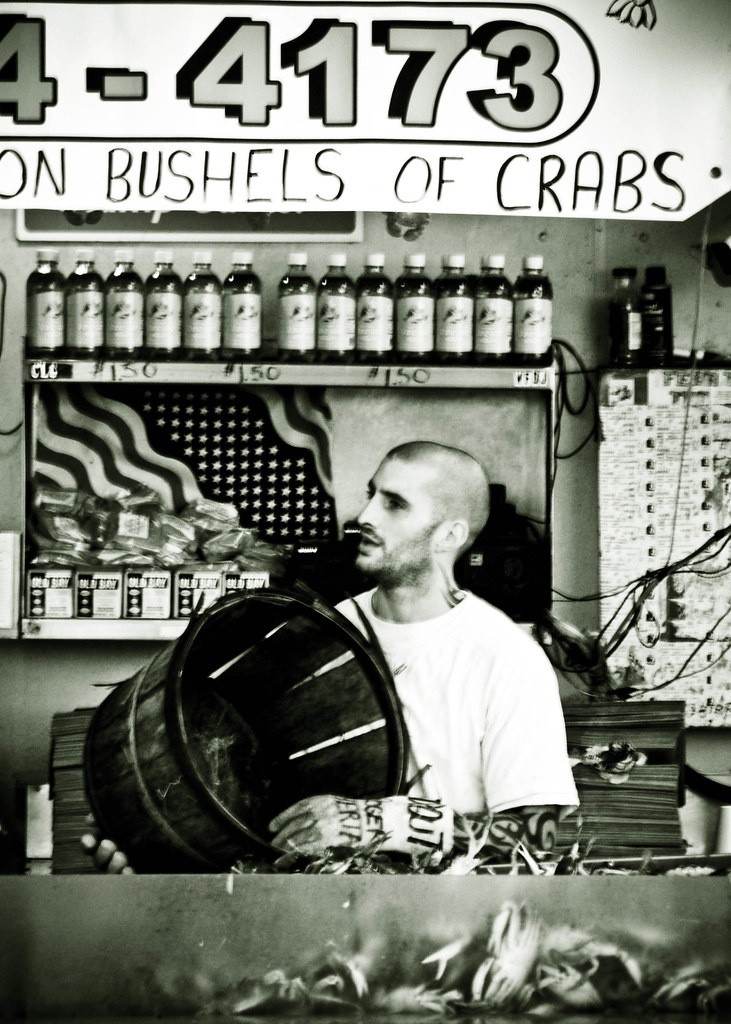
[81,587,407,874]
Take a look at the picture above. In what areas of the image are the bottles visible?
[24,248,555,368]
[609,265,642,371]
[642,265,675,370]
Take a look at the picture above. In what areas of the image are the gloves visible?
[270,795,453,867]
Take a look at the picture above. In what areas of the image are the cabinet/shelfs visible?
[20,355,561,649]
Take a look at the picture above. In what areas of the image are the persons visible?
[80,442,579,874]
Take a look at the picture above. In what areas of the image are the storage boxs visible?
[26,561,270,621]
